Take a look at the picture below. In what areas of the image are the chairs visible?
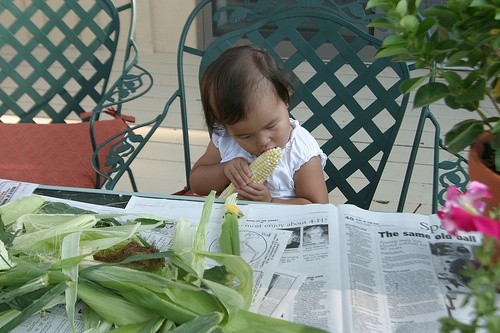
[0,0,469,215]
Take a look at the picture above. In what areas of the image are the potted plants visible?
[365,0,500,266]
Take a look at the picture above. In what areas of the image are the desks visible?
[1,177,500,333]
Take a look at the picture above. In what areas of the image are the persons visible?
[189,44,330,204]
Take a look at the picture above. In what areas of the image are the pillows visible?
[0,105,136,189]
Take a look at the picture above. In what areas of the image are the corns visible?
[217,147,281,199]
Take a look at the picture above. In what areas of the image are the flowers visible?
[435,181,499,240]
